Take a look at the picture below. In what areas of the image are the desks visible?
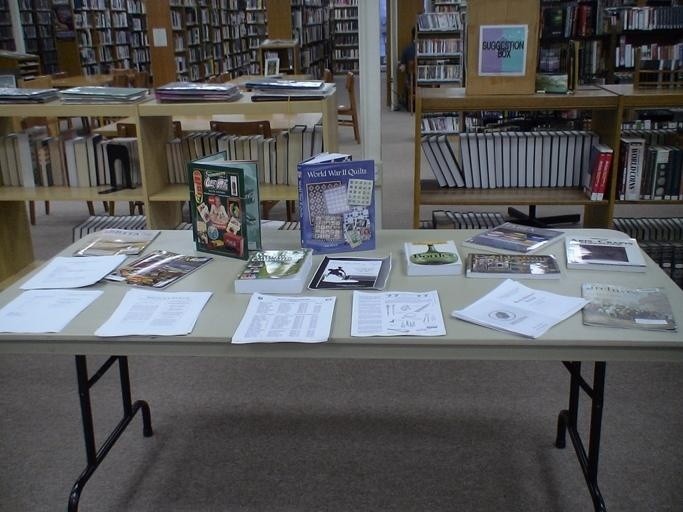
[51,75,113,127]
[0,227,682,512]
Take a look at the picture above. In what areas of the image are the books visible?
[294,150,377,255]
[0,77,338,232]
[71,229,215,291]
[2,0,360,84]
[402,127,683,286]
[413,0,683,135]
[234,248,314,294]
[580,281,677,332]
[186,148,262,260]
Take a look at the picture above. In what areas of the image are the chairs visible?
[17,75,53,127]
[103,69,129,120]
[134,71,150,88]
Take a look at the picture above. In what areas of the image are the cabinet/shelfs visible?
[414,11,465,143]
[265,0,335,81]
[0,1,58,74]
[597,84,683,288]
[0,81,339,294]
[244,0,265,75]
[51,0,153,81]
[329,0,359,75]
[144,0,268,86]
[259,39,300,75]
[538,0,682,86]
[414,86,619,229]
